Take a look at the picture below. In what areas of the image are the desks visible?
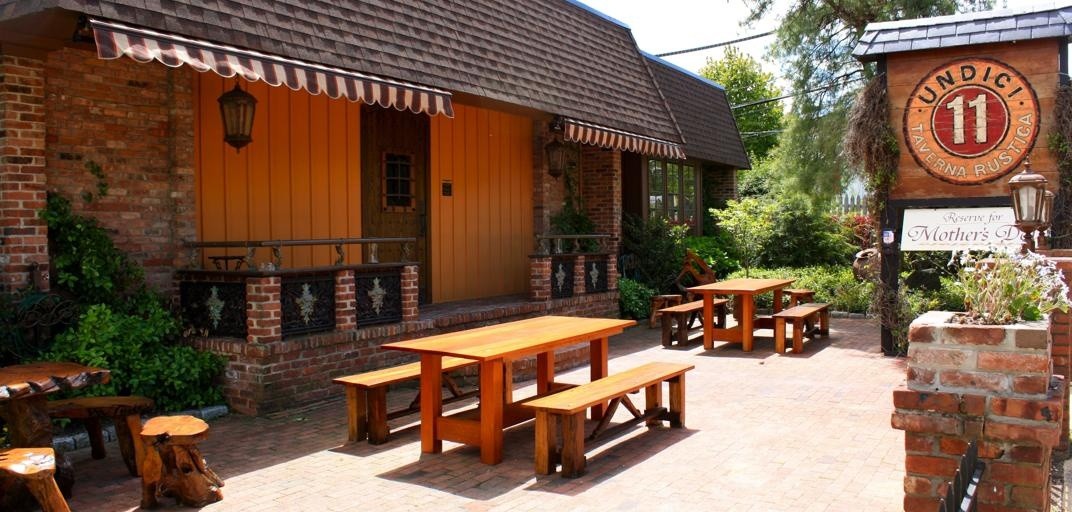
[379,313,638,467]
[0,362,111,500]
[683,277,794,352]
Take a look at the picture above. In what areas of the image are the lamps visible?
[543,115,567,181]
[216,72,259,156]
[1007,152,1048,255]
[1035,190,1057,246]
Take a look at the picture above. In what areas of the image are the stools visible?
[46,396,156,477]
[649,294,683,329]
[1,446,70,512]
[139,414,225,510]
[782,288,816,324]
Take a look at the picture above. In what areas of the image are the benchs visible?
[656,298,730,347]
[772,302,833,354]
[331,355,513,446]
[519,360,695,479]
